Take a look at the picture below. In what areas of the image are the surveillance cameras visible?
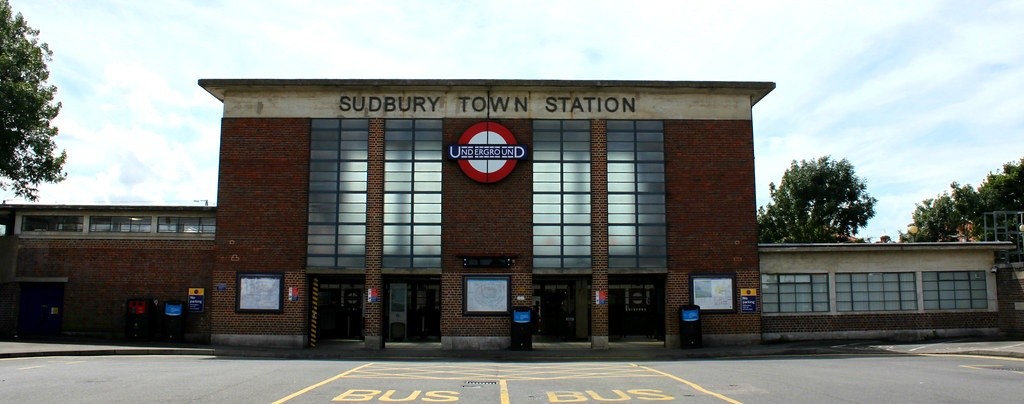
[991,267,998,273]
